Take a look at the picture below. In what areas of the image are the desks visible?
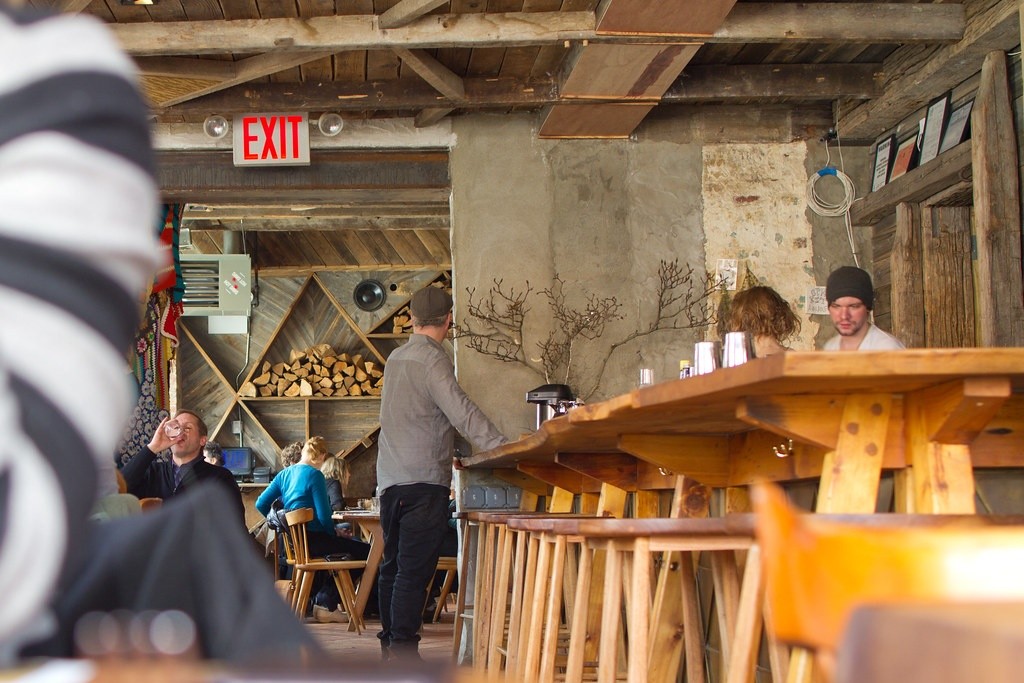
[330,514,386,632]
[460,347,1024,683]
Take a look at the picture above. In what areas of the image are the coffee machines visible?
[525,382,573,432]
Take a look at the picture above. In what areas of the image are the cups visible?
[721,331,748,367]
[679,360,690,379]
[372,496,380,513]
[639,368,653,389]
[694,342,716,375]
[164,421,181,438]
[690,367,694,378]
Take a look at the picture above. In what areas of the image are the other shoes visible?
[419,605,441,621]
[312,604,348,623]
[381,645,429,669]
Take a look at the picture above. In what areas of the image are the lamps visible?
[203,111,344,167]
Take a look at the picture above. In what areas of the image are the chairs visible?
[282,508,366,636]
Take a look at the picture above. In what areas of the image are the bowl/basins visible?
[357,498,371,508]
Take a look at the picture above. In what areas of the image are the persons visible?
[821,266,906,351]
[724,286,802,360]
[0,0,531,683]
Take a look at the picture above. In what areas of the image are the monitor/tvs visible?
[221,446,255,476]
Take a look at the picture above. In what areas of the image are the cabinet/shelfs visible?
[168,270,452,487]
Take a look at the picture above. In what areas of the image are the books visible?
[333,510,370,514]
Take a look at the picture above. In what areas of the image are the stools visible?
[452,510,1024,683]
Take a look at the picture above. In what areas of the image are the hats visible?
[826,267,874,311]
[412,286,453,320]
